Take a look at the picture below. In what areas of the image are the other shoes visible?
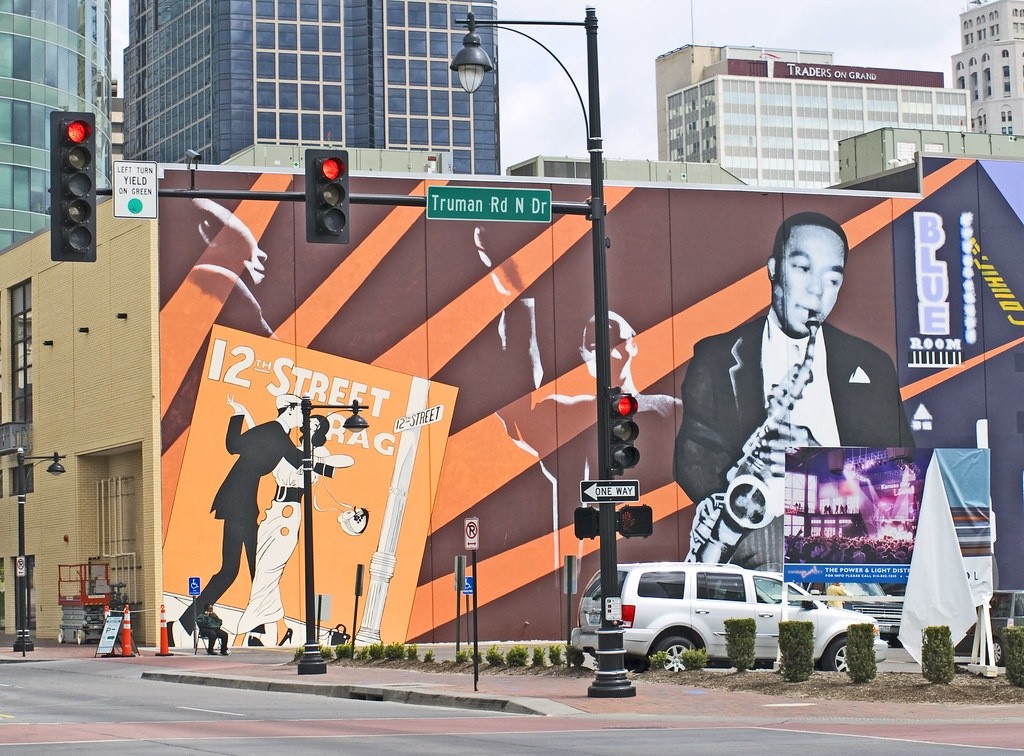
[221,652,228,655]
[207,649,217,655]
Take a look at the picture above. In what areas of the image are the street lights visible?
[13,447,67,652]
[447,11,642,699]
[299,392,372,675]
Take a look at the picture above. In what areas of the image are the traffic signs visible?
[579,480,641,503]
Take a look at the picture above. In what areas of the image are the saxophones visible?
[678,310,824,565]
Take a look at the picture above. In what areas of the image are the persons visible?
[197,603,229,655]
[671,211,916,570]
[827,582,847,608]
[784,503,915,564]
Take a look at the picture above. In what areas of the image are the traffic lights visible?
[619,505,653,539]
[609,388,641,470]
[573,506,599,539]
[304,147,353,242]
[49,110,99,261]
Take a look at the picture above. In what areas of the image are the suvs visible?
[961,590,1024,667]
[570,560,888,673]
[806,574,909,648]
